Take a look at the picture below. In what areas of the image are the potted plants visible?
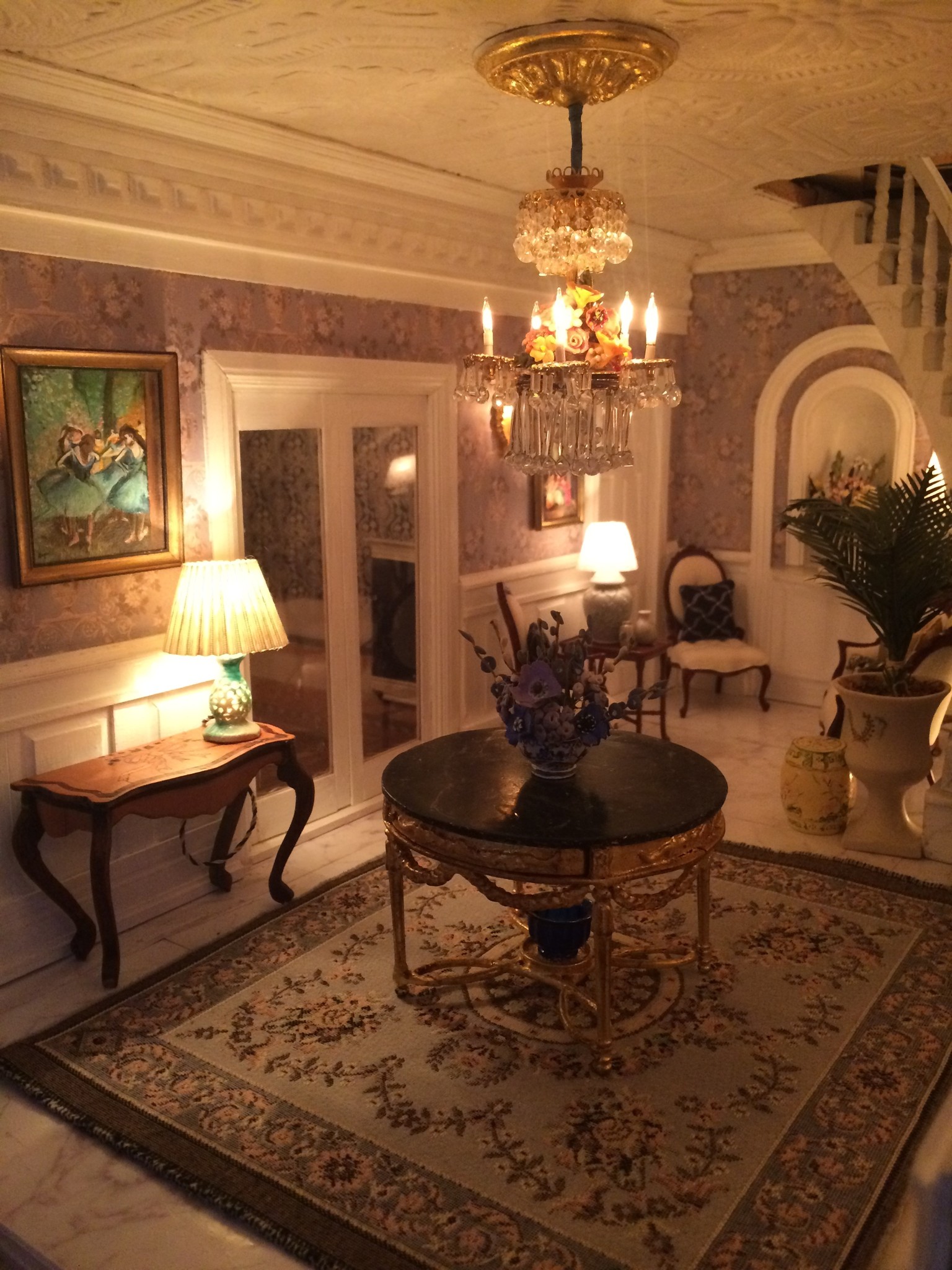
[777,451,952,859]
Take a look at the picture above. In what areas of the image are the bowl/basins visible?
[526,898,594,961]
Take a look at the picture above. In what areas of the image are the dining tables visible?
[658,543,773,718]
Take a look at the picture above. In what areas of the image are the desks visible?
[561,625,675,742]
[9,719,314,987]
[378,725,730,1074]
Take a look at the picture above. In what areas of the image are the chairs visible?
[821,611,952,786]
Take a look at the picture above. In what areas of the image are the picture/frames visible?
[529,469,587,531]
[0,344,188,591]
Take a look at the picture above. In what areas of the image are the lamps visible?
[460,13,683,475]
[579,521,640,644]
[161,557,289,741]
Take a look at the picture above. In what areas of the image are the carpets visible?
[2,841,952,1270]
